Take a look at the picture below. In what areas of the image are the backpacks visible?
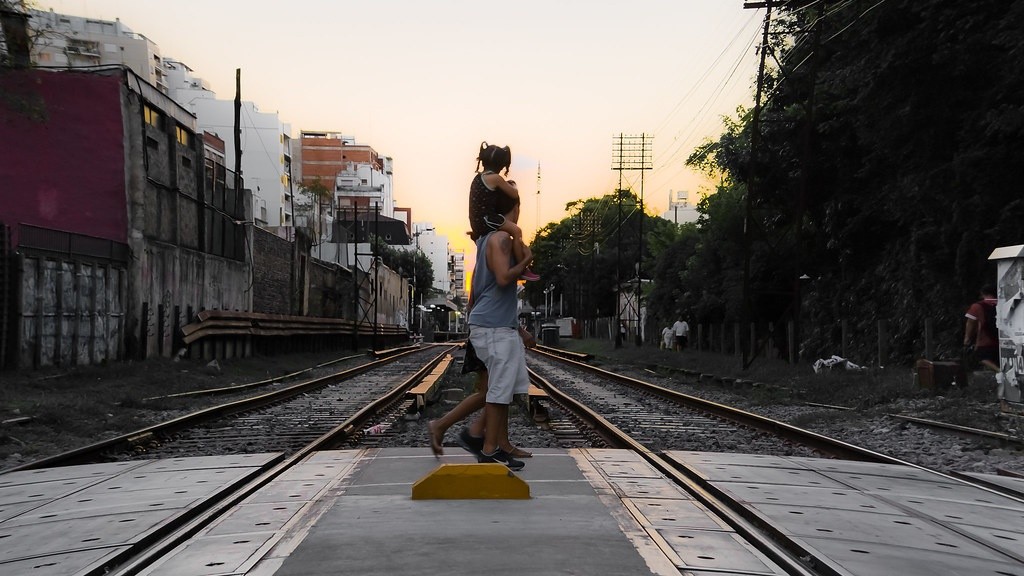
[977,302,998,344]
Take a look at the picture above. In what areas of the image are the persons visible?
[962,282,999,374]
[427,140,542,471]
[620,320,628,342]
[661,316,690,352]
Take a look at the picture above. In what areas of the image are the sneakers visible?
[520,270,540,281]
[478,445,525,471]
[459,427,485,456]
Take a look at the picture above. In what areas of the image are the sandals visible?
[508,448,533,458]
[427,420,443,455]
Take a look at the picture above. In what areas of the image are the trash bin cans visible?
[541,322,561,346]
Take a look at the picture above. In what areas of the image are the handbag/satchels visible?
[660,342,666,349]
